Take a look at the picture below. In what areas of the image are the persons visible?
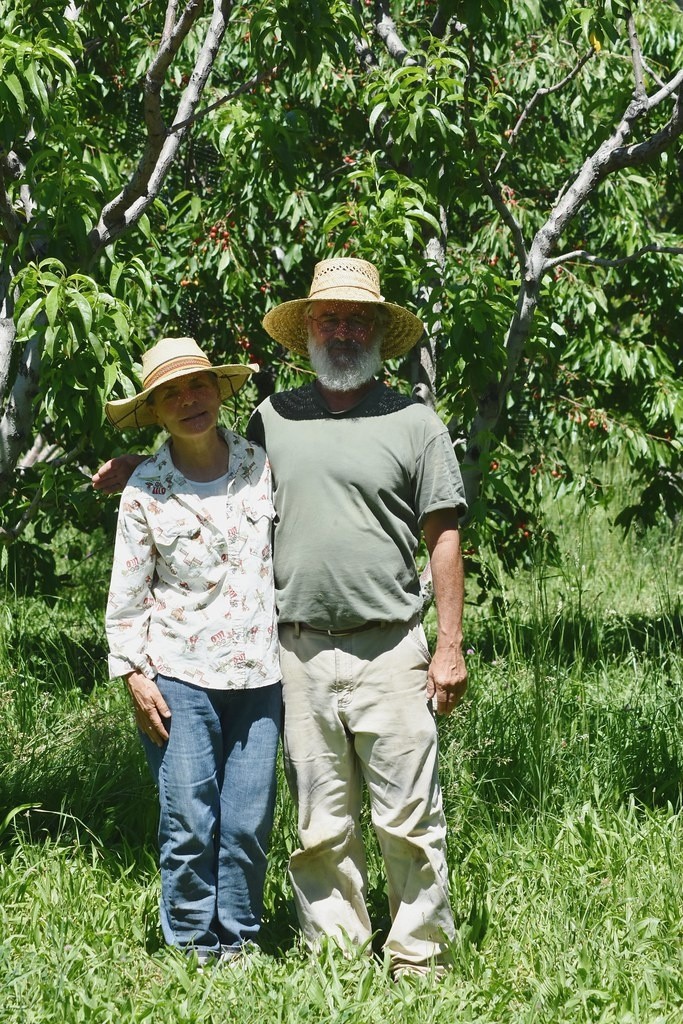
[101,335,283,966]
[91,257,482,989]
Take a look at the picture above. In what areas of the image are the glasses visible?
[308,313,379,332]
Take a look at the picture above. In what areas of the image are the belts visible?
[285,621,382,637]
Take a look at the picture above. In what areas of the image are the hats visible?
[261,257,424,363]
[104,337,261,430]
